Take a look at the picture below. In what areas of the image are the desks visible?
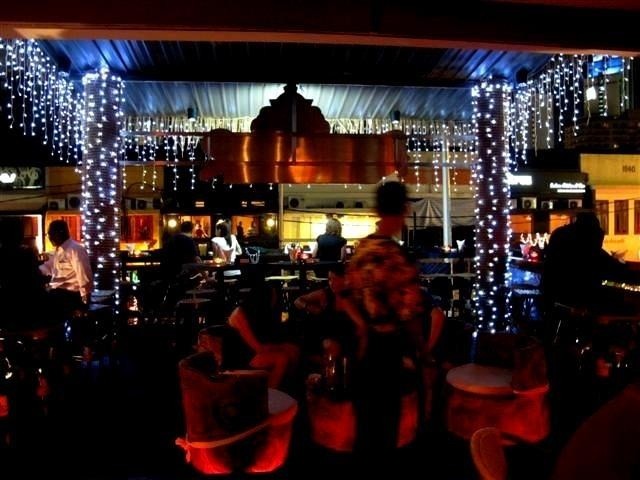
[306,373,426,452]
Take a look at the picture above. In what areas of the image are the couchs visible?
[212,306,301,389]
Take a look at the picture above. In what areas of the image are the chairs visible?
[174,350,298,476]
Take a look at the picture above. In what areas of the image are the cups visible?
[222,247,235,265]
[286,248,298,264]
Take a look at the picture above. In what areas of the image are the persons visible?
[541,212,640,316]
[2,217,91,334]
[161,219,243,286]
[219,180,445,378]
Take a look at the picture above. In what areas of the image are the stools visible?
[447,364,552,447]
